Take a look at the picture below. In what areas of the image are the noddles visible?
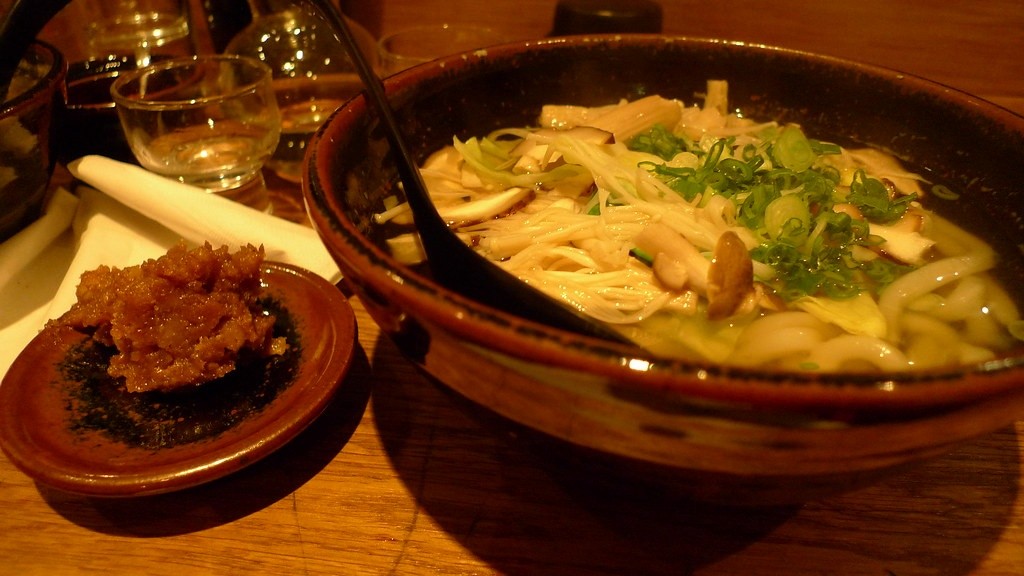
[373,78,1024,370]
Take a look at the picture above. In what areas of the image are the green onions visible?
[588,118,1023,369]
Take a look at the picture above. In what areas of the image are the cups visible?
[376,24,517,76]
[0,39,63,240]
[79,0,187,69]
[112,54,280,217]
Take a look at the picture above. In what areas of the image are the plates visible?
[1,261,357,497]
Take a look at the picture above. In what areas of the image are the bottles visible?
[223,0,380,222]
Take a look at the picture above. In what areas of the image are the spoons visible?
[289,0,644,349]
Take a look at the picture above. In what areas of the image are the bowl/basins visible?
[304,36,1024,475]
[51,51,202,167]
